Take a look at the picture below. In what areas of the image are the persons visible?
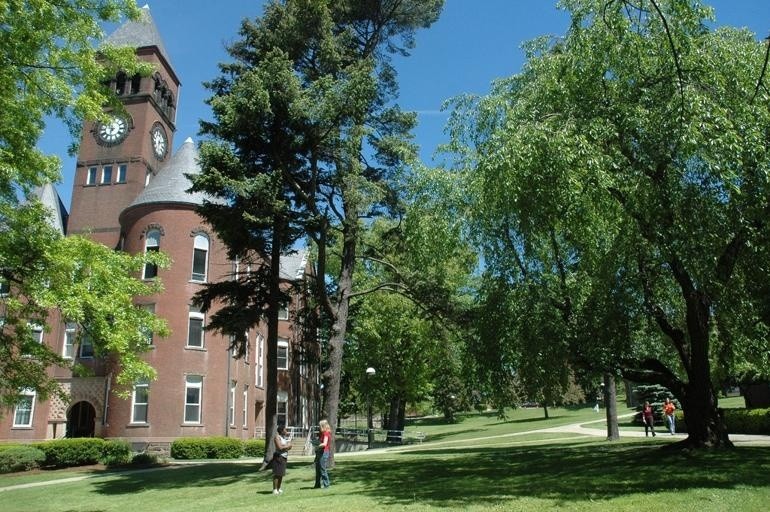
[311,419,333,488]
[663,395,677,436]
[270,424,293,495]
[640,399,658,437]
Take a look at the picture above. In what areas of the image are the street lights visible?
[365,367,376,448]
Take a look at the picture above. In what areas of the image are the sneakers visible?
[273,488,283,495]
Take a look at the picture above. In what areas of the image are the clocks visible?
[97,114,128,143]
[151,127,167,158]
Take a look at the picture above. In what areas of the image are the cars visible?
[521,401,543,408]
